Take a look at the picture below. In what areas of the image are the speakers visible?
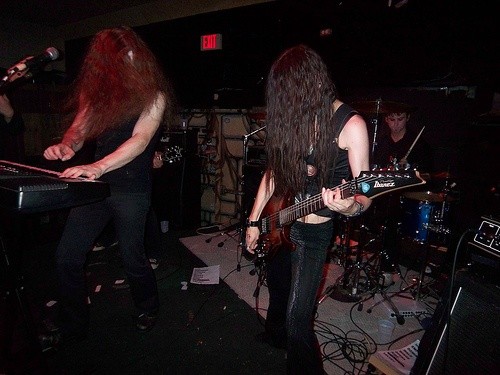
[409,267,500,375]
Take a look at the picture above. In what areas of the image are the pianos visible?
[0,158,111,360]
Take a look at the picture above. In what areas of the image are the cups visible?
[378,320,394,344]
[160,221,168,232]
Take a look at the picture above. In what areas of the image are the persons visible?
[374,108,425,270]
[243,46,372,375]
[0,27,171,350]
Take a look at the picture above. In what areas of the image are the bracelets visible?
[245,219,261,227]
[348,203,363,218]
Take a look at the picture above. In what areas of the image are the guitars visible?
[256,156,426,252]
[0,58,33,89]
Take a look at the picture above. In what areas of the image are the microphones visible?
[12,48,60,72]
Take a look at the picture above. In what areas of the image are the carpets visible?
[178,229,433,375]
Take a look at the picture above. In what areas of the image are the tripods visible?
[320,120,405,325]
[206,137,257,272]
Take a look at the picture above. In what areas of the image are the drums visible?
[398,191,455,244]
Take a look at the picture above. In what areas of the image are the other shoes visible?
[136,314,154,333]
[64,335,87,344]
[255,331,269,341]
[379,273,392,286]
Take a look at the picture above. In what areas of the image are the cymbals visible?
[349,100,412,114]
[431,172,461,178]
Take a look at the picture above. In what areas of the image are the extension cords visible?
[368,356,399,375]
